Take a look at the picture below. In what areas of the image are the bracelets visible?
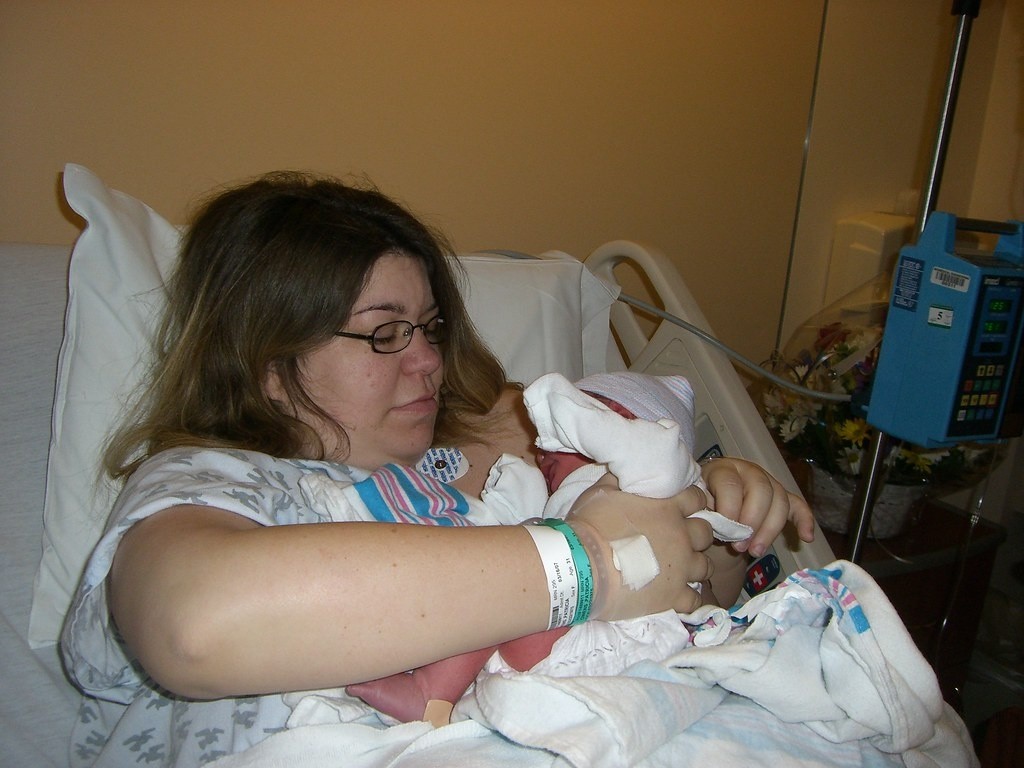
[524,523,578,632]
[538,517,593,627]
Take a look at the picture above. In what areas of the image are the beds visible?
[0,163,982,768]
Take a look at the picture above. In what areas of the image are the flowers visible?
[758,319,1000,492]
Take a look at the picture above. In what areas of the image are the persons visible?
[58,170,814,768]
[347,372,706,722]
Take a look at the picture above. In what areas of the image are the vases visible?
[807,455,920,539]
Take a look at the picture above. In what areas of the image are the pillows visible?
[27,162,622,650]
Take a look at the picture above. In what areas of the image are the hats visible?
[573,371,696,457]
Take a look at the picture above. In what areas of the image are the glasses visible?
[333,308,450,354]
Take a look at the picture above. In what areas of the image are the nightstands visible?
[818,495,1007,717]
